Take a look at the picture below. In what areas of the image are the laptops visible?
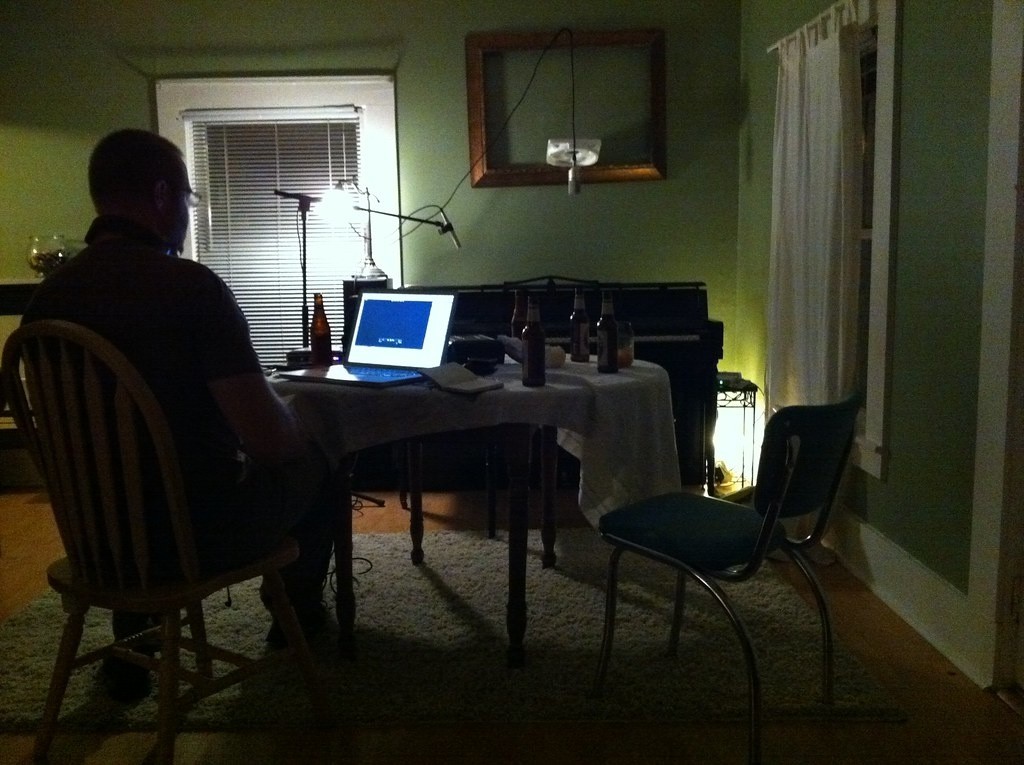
[279,288,459,389]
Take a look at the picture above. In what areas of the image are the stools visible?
[716,387,758,489]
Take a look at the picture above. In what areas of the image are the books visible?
[415,361,504,396]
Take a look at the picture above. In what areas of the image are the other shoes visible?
[267,605,335,642]
[105,651,145,674]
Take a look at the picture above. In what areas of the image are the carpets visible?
[0,528,909,735]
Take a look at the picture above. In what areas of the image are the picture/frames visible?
[464,26,669,186]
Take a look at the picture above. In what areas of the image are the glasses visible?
[171,183,201,206]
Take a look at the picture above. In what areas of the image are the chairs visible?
[588,389,864,765]
[1,319,330,765]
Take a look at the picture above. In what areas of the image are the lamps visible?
[546,137,603,198]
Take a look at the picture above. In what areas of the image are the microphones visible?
[440,208,461,249]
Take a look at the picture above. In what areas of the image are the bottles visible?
[616,320,634,368]
[26,234,69,277]
[510,289,529,339]
[596,290,619,374]
[310,292,333,367]
[521,294,548,387]
[570,286,591,363]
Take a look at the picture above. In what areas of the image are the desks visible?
[258,354,670,671]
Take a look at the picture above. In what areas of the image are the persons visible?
[19,129,336,704]
